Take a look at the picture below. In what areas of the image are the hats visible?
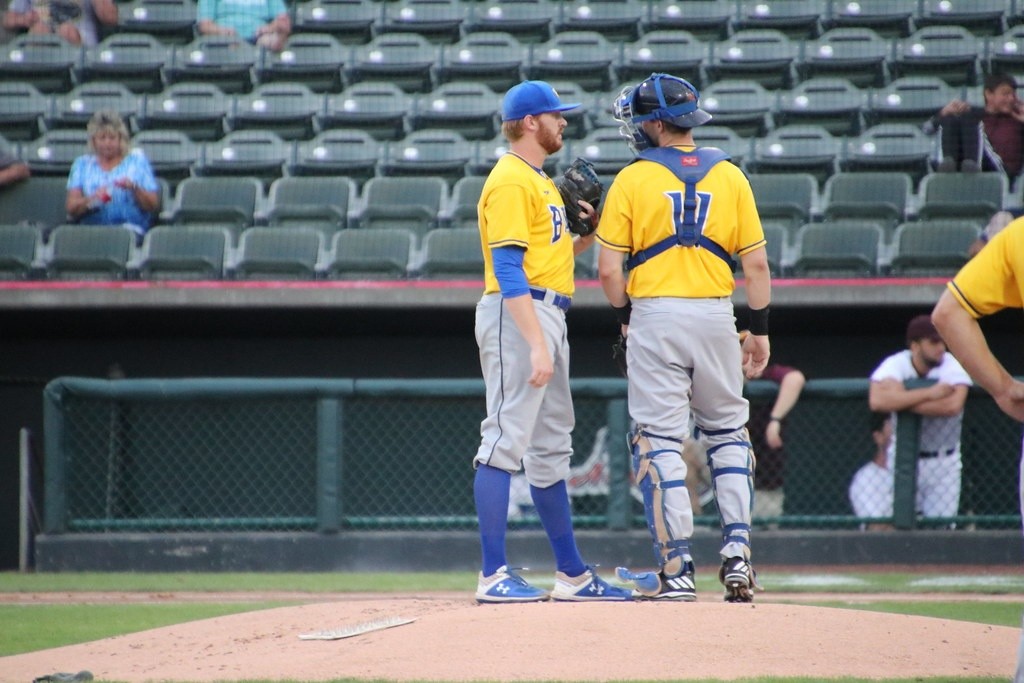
[908,315,943,340]
[739,329,751,341]
[501,80,583,122]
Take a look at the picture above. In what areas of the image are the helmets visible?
[633,78,712,128]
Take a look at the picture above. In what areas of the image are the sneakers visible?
[552,565,636,600]
[475,565,551,604]
[721,557,753,602]
[632,570,697,602]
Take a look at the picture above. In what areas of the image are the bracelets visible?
[767,416,784,424]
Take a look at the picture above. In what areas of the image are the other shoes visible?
[936,155,982,174]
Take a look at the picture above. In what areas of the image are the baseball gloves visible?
[610,333,627,378]
[556,156,606,237]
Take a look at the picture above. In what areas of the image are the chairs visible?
[0,0,1024,280]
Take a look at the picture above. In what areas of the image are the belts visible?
[917,448,955,458]
[529,290,571,312]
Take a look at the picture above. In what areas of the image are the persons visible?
[0,136,29,187]
[849,407,898,532]
[475,80,636,600]
[922,72,1024,193]
[930,215,1022,424]
[66,108,159,243]
[194,0,291,53]
[3,0,117,47]
[868,316,975,530]
[594,74,771,603]
[687,326,805,531]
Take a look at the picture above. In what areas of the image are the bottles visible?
[95,179,119,208]
